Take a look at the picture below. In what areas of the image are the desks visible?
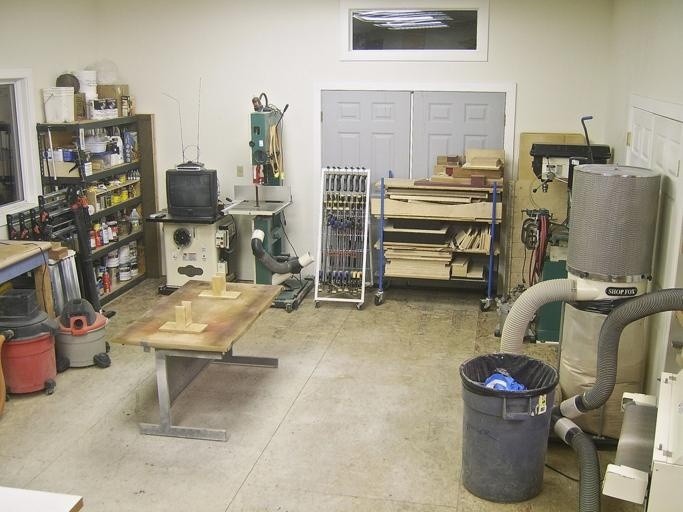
[109,280,285,440]
[0,239,57,320]
[0,486,84,512]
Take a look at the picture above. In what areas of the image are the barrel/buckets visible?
[41,86,76,126]
[54,315,110,367]
[0,335,57,395]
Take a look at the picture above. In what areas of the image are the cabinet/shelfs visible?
[8,114,159,308]
[310,167,505,311]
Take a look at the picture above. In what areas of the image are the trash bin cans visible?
[460,353,560,503]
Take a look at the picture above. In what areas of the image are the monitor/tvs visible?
[166,169,218,216]
